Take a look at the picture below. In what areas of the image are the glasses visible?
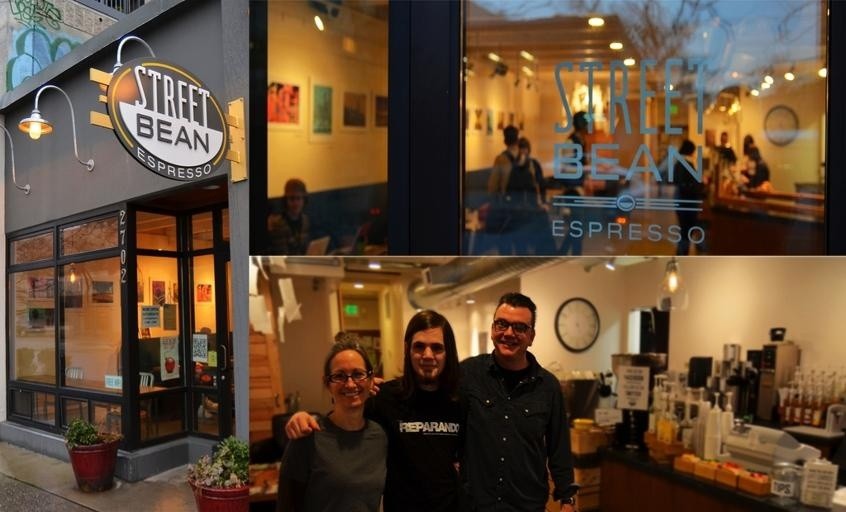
[493,319,529,332]
[325,370,372,382]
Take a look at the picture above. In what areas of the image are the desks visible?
[15,374,167,448]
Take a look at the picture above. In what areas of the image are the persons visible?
[285,310,471,512]
[487,112,771,255]
[371,292,579,511]
[267,180,310,255]
[277,337,389,512]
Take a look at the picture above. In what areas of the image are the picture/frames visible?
[268,70,533,144]
[22,274,212,333]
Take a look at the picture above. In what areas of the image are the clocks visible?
[553,297,599,353]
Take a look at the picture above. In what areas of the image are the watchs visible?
[561,497,576,504]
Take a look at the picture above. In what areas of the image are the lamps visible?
[654,258,690,313]
[17,83,95,173]
[461,36,533,89]
[583,257,617,272]
[63,234,86,298]
[97,35,155,92]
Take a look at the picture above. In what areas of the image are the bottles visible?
[647,390,733,463]
[775,392,841,426]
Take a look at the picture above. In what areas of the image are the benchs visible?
[266,164,499,255]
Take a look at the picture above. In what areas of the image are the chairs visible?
[63,366,86,420]
[105,370,156,443]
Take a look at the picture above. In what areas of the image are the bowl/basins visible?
[574,418,593,431]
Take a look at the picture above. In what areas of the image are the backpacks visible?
[507,163,537,210]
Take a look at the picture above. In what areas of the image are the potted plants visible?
[60,417,121,493]
[184,432,251,512]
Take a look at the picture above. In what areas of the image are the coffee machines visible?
[729,342,801,425]
